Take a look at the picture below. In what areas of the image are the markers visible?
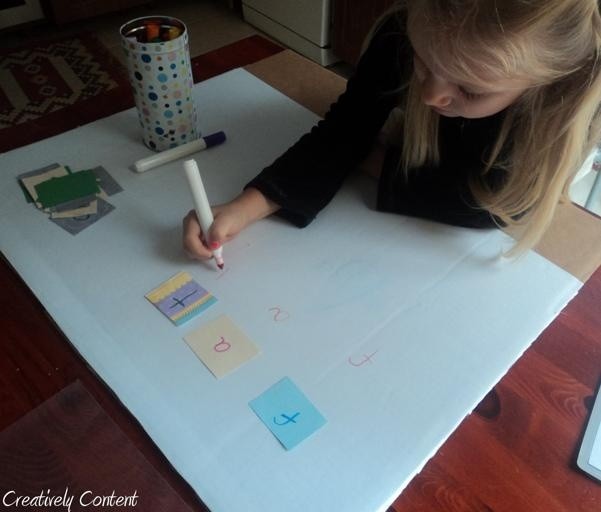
[133,130,227,173]
[183,158,225,270]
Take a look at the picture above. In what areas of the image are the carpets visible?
[0,13,131,153]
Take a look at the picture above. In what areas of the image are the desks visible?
[0,33,600,512]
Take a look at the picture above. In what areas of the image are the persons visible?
[177,2,600,265]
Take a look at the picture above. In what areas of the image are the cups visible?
[117,14,199,153]
